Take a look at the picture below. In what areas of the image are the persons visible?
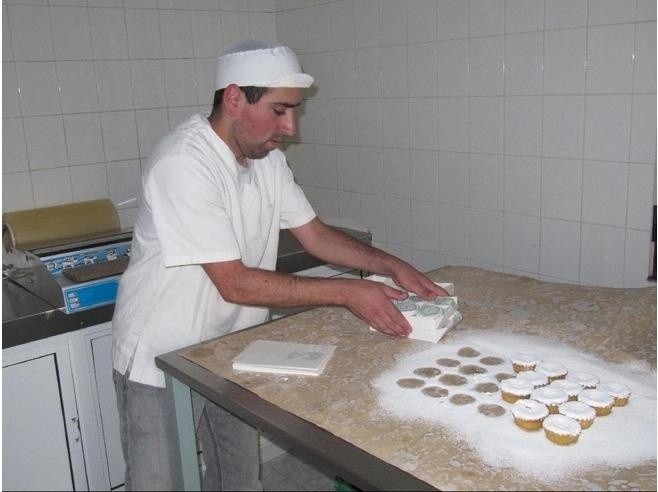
[109,47,450,491]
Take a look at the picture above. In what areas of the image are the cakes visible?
[531,387,570,416]
[500,378,532,403]
[597,382,632,407]
[517,370,549,387]
[508,352,538,373]
[558,402,597,428]
[535,361,568,382]
[551,378,583,403]
[510,398,549,431]
[566,371,599,388]
[576,388,614,416]
[542,413,581,446]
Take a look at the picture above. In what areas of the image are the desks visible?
[155,264,657,492]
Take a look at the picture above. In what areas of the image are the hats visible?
[215,45,314,90]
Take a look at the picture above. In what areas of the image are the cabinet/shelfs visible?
[2,262,369,492]
